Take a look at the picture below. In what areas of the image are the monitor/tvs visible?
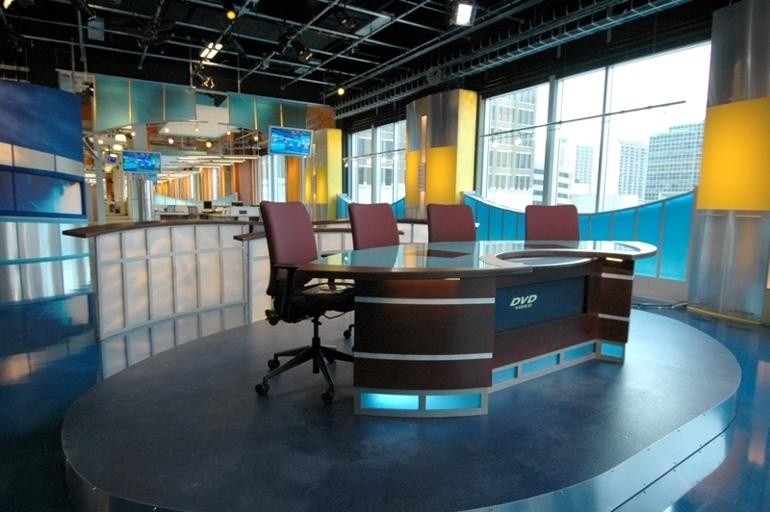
[266,124,313,159]
[121,147,163,174]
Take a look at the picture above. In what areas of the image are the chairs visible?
[348,198,581,250]
[253,199,358,403]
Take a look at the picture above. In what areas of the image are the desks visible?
[292,236,659,427]
